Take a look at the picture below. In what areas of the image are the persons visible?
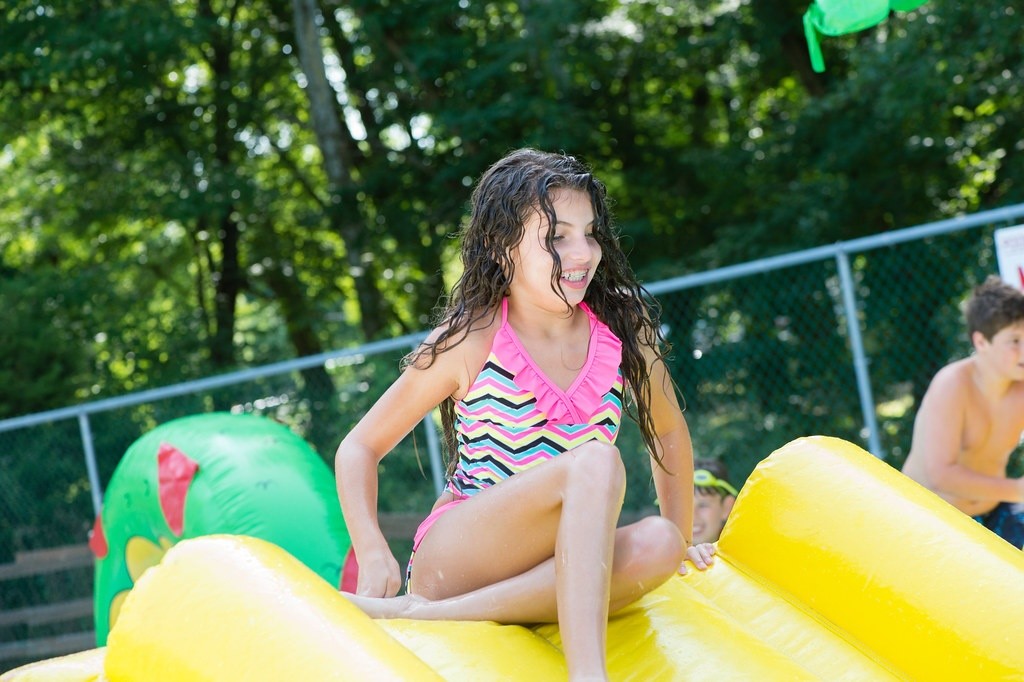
[901,277,1024,551]
[336,147,714,682]
[653,459,734,545]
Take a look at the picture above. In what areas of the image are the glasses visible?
[653,469,738,506]
